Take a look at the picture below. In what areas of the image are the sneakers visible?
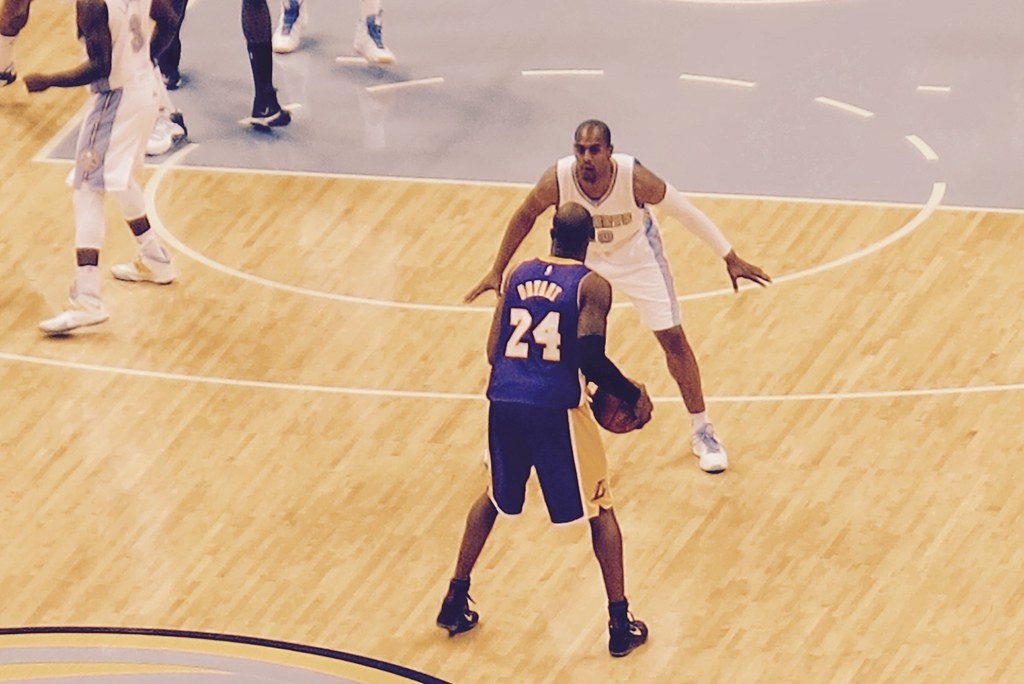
[110,249,174,284]
[354,16,395,65]
[694,422,727,472]
[252,90,289,128]
[273,2,306,54]
[609,620,648,656]
[143,110,187,156]
[37,294,108,334]
[157,62,182,88]
[436,599,478,633]
[0,62,16,88]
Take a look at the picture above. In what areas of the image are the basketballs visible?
[592,385,651,434]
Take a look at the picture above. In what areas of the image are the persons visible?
[271,0,396,62]
[146,0,291,155]
[0,0,33,88]
[464,119,772,474]
[438,204,653,657]
[22,0,176,331]
